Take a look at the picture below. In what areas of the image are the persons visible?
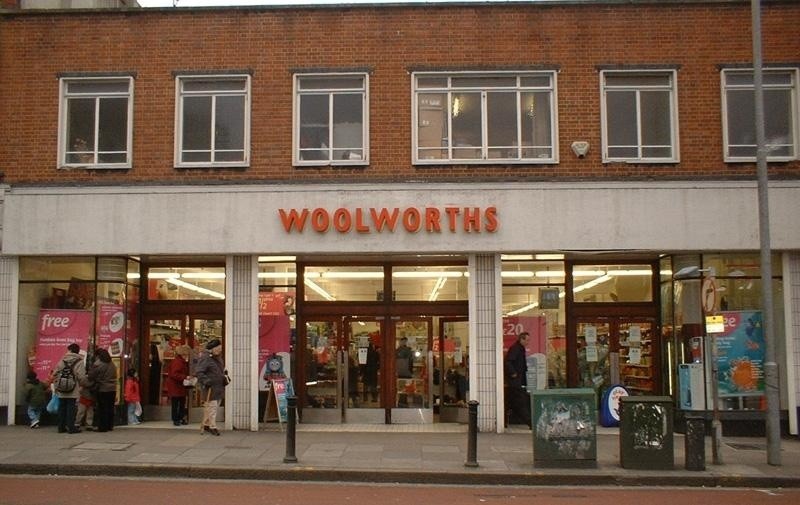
[25,344,117,433]
[124,368,144,425]
[167,346,194,426]
[195,338,225,436]
[396,337,413,408]
[505,332,532,430]
[359,342,380,402]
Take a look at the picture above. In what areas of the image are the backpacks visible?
[53,358,81,393]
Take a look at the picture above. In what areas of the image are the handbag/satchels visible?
[224,375,231,385]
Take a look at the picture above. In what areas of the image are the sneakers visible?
[58,424,99,434]
[127,421,141,426]
[174,419,187,425]
[203,426,219,436]
[31,420,40,430]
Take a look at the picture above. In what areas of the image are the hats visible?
[205,339,221,350]
[25,370,36,380]
[175,345,194,355]
